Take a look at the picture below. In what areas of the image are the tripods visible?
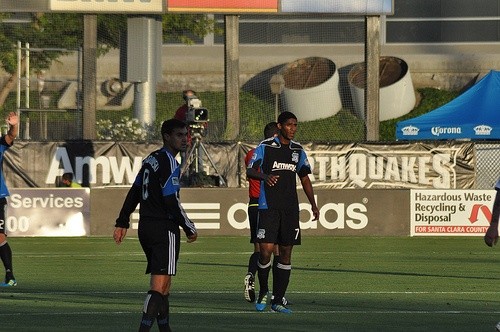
[179,124,227,187]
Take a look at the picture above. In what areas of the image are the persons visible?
[485,173,500,247]
[0,111,21,288]
[244,112,321,313]
[115,120,198,332]
[59,172,74,187]
[174,91,207,174]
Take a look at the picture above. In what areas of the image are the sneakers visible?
[244,272,256,304]
[271,304,293,314]
[270,293,288,305]
[0,279,17,287]
[256,290,269,311]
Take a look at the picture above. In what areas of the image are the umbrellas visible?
[397,70,500,190]
